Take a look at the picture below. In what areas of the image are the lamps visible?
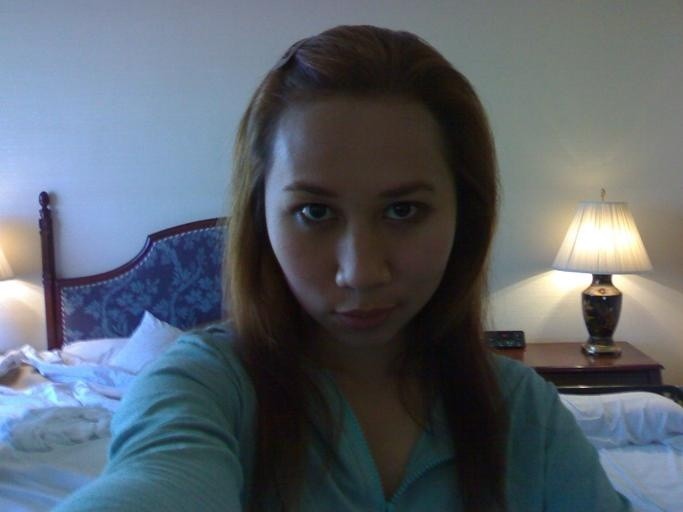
[550,189,654,357]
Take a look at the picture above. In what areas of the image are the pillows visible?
[110,309,183,376]
[559,391,683,450]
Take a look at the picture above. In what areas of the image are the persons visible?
[53,24,636,512]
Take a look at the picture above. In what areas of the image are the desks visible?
[481,336,664,398]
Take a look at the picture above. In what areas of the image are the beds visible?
[0,191,234,510]
[552,383,683,512]
